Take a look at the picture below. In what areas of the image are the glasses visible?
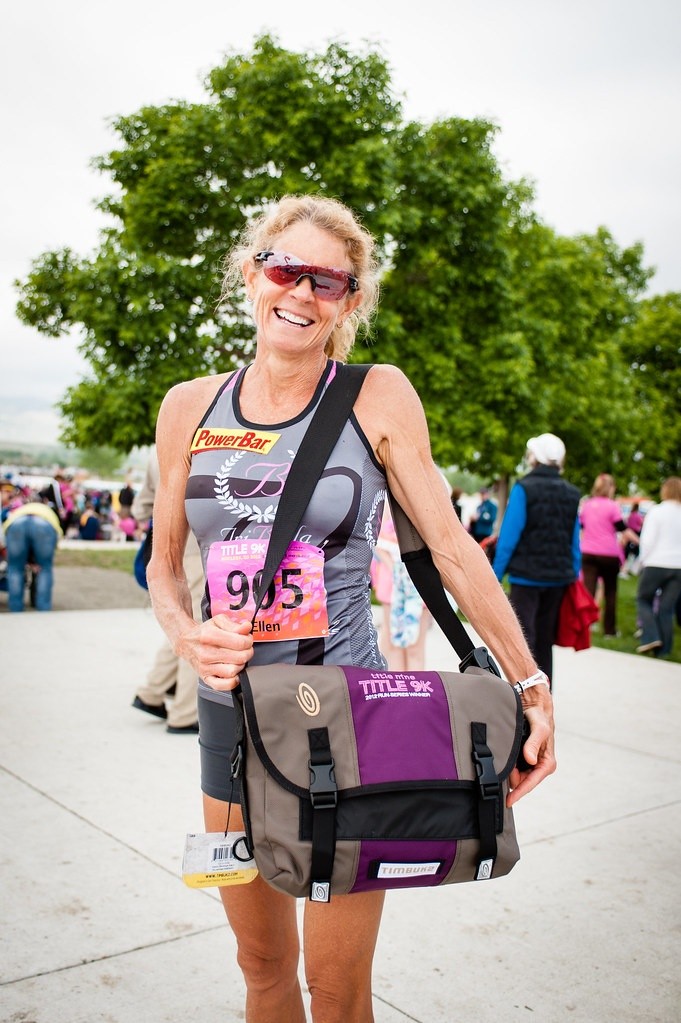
[254,251,359,301]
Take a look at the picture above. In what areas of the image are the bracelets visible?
[513,670,550,693]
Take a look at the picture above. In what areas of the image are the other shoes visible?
[635,640,663,653]
[167,721,199,733]
[132,696,167,719]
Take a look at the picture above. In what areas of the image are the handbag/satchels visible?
[230,663,523,904]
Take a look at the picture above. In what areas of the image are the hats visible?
[526,433,565,465]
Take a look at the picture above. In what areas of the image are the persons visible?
[147,193,557,1023]
[619,503,643,580]
[130,446,206,734]
[579,474,640,637]
[451,487,496,565]
[373,501,430,672]
[636,476,681,661]
[3,502,64,611]
[0,468,149,592]
[493,433,582,693]
